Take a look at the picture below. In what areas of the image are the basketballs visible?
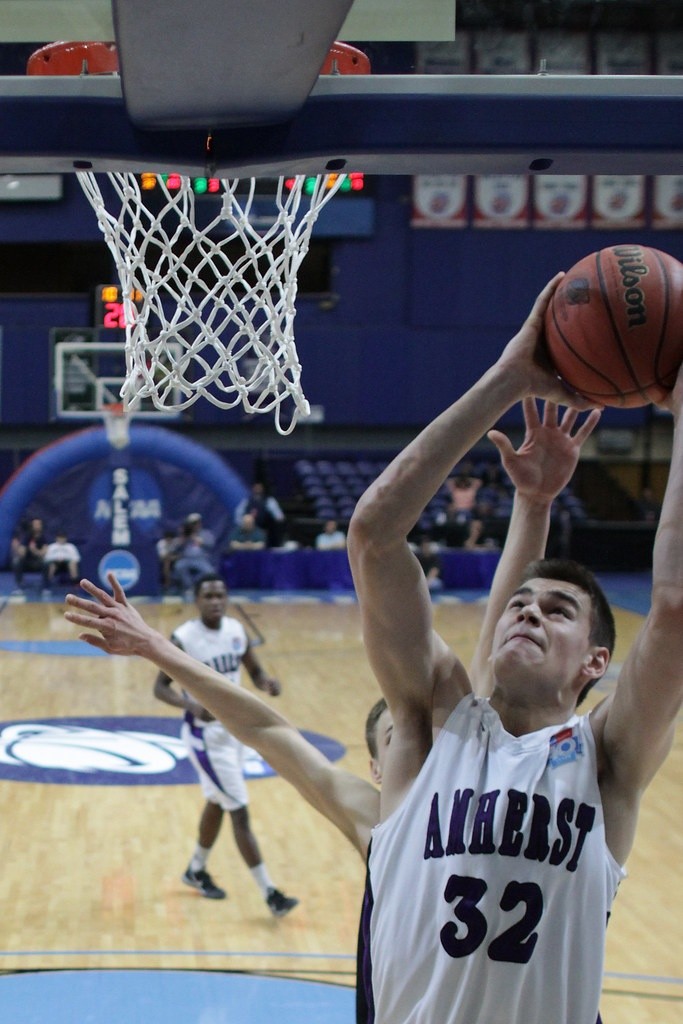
[542,243,683,410]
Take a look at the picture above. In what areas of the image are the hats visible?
[187,513,201,524]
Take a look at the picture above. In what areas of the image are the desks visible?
[221,548,501,592]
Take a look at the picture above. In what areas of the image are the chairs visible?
[295,457,588,524]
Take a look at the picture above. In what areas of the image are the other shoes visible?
[8,589,24,596]
[42,587,53,600]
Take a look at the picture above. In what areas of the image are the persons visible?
[10,271,682,1024]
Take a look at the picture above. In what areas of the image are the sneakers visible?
[181,867,226,898]
[266,889,298,916]
